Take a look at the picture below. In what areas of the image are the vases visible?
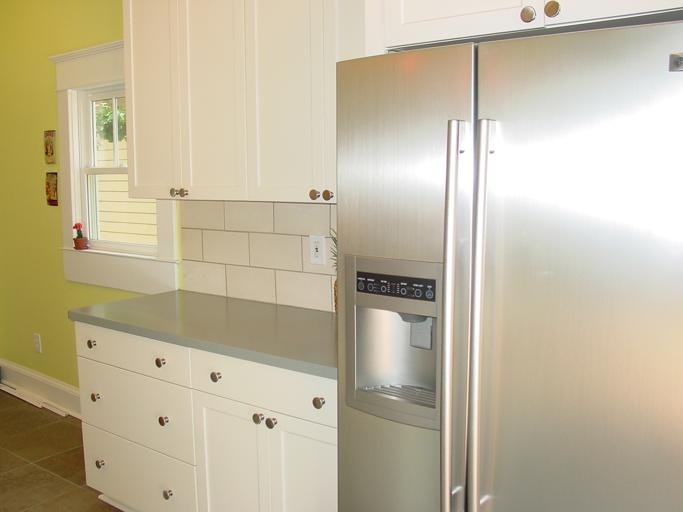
[73,237,89,251]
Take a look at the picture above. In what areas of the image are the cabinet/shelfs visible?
[123,2,248,203]
[249,0,364,204]
[189,346,338,512]
[74,321,197,512]
[364,2,683,49]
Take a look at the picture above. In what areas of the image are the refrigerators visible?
[338,21,683,512]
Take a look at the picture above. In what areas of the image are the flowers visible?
[72,223,82,238]
